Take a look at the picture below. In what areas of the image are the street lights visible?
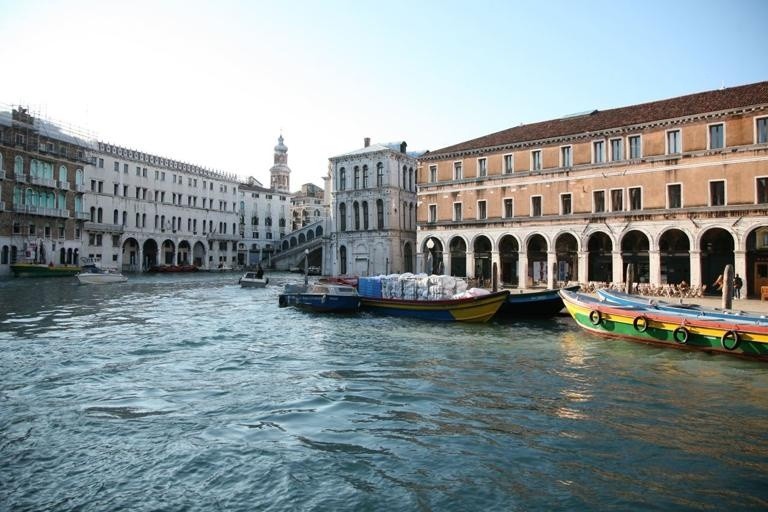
[425,238,434,274]
[304,248,309,284]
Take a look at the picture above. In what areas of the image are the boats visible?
[9,263,82,278]
[239,272,266,288]
[77,272,128,285]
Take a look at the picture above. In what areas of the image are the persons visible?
[679,280,689,292]
[734,274,742,299]
[255,266,264,279]
